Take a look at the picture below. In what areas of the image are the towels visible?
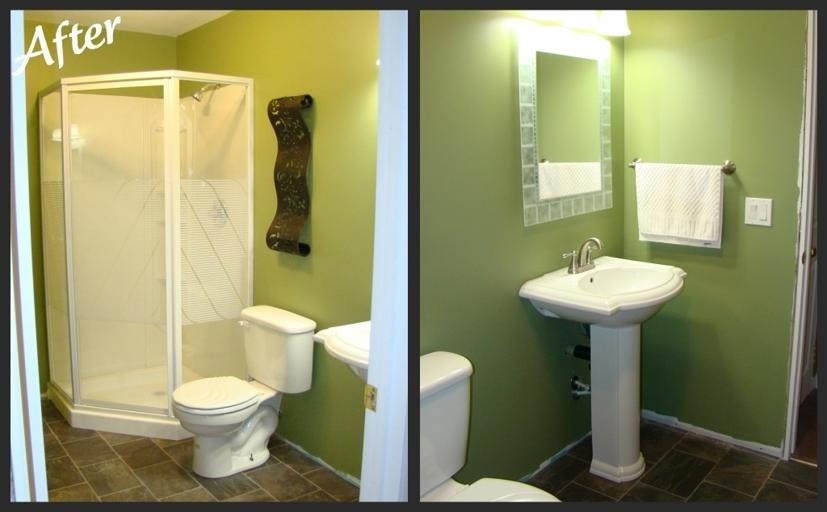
[635,162,724,251]
[538,162,601,200]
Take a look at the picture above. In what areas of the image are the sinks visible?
[315,319,371,377]
[519,255,688,327]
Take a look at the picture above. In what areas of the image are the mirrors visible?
[517,39,613,228]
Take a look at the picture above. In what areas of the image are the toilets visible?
[168,306,314,477]
[419,351,559,503]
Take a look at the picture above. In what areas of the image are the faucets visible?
[577,236,603,268]
